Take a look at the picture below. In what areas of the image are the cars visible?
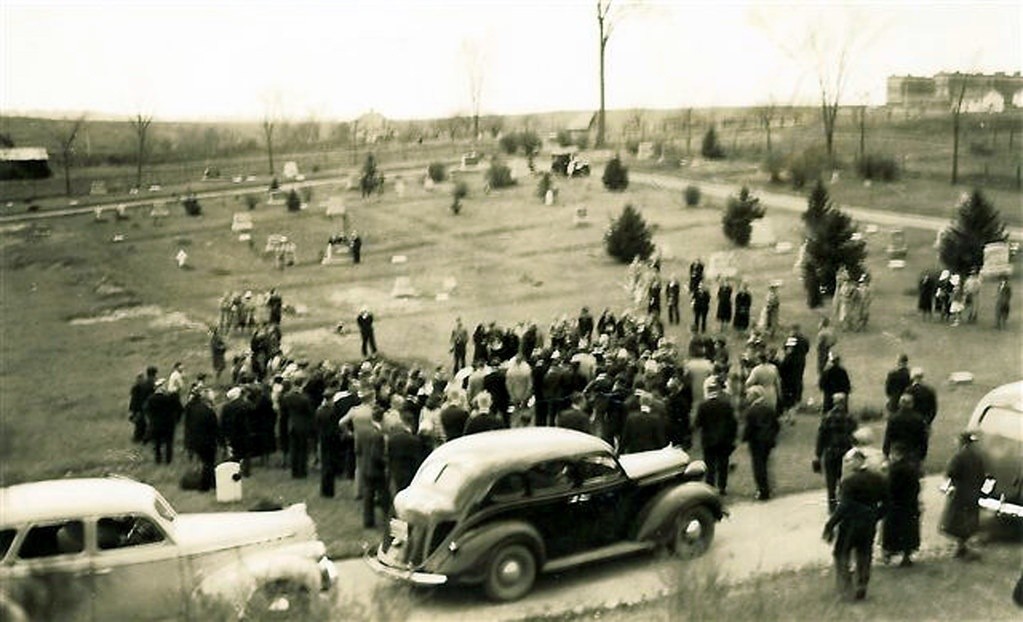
[364,425,729,603]
[0,475,340,622]
[940,379,1023,528]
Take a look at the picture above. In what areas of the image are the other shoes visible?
[900,558,912,566]
[856,586,867,600]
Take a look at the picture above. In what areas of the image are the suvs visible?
[551,150,589,178]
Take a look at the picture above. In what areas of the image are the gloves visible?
[812,459,822,475]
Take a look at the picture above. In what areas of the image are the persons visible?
[917,267,982,327]
[448,312,470,375]
[832,268,872,332]
[686,255,713,333]
[126,307,810,531]
[646,261,662,321]
[759,282,780,334]
[329,230,362,264]
[714,274,733,331]
[938,432,986,558]
[356,305,377,357]
[359,172,385,202]
[816,316,939,600]
[664,272,680,326]
[991,273,1011,329]
[733,283,754,338]
[219,286,282,335]
[802,262,822,308]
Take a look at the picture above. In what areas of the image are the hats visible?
[226,387,242,400]
[853,427,872,445]
